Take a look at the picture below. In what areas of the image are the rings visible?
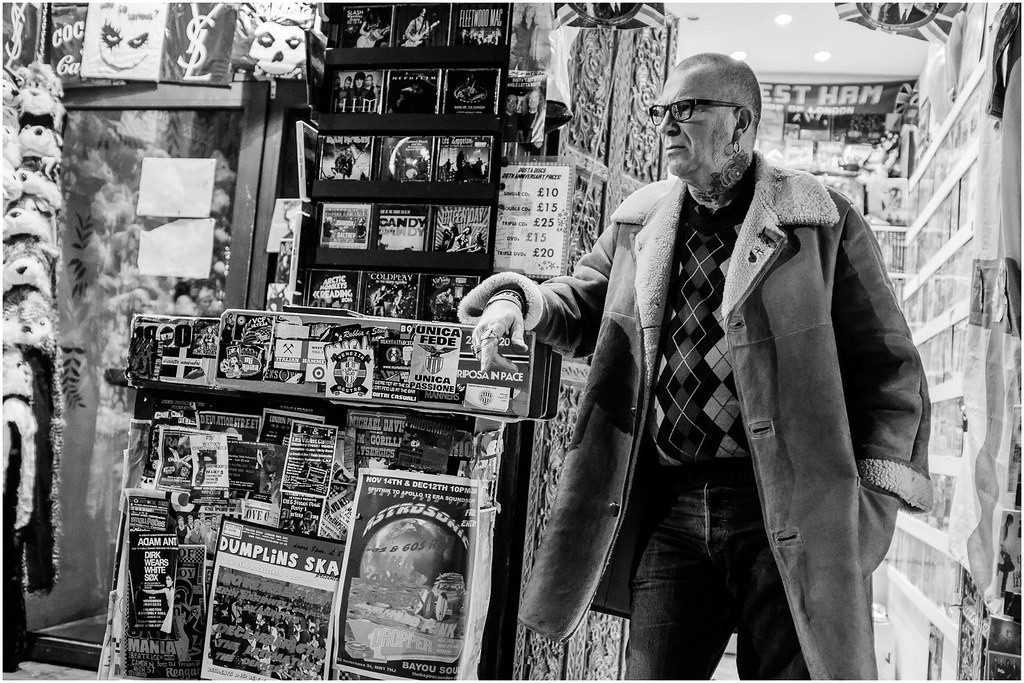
[480,328,501,340]
[471,343,482,355]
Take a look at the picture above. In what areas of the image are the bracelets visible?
[485,289,526,316]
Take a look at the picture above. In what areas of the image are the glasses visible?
[649,99,757,126]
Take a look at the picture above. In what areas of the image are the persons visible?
[458,52,934,681]
